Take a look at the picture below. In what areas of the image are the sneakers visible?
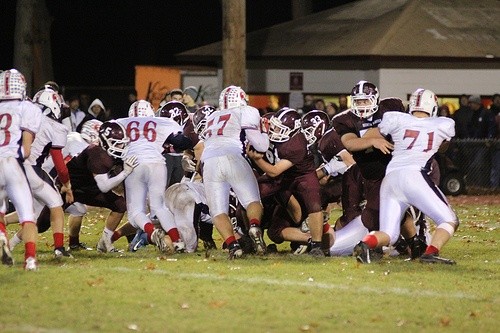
[172,239,185,254]
[97,239,124,253]
[24,257,36,271]
[206,222,457,265]
[130,229,149,253]
[0,231,14,266]
[54,248,74,259]
[66,244,93,252]
[152,228,175,254]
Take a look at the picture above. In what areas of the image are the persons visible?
[301,80,440,259]
[110,89,218,251]
[227,93,335,257]
[0,68,139,272]
[201,85,269,260]
[355,88,460,266]
[437,92,500,190]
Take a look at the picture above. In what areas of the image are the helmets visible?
[0,69,27,100]
[408,88,439,117]
[32,88,62,120]
[98,120,130,158]
[129,100,156,117]
[218,86,249,109]
[301,109,331,147]
[160,102,190,124]
[80,119,104,146]
[269,107,302,142]
[263,112,276,132]
[192,105,217,135]
[350,80,380,117]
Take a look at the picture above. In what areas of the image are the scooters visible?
[435,153,465,196]
